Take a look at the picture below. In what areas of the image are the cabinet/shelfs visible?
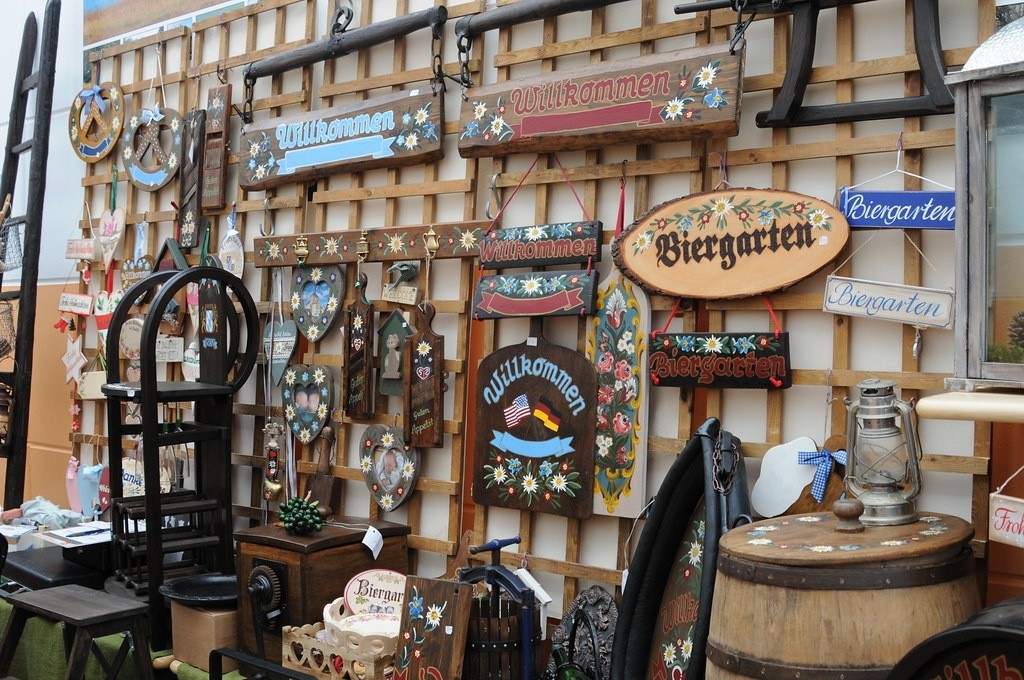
[944,58,1024,393]
[101,267,261,649]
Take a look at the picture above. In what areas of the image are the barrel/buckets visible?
[705,504,982,680]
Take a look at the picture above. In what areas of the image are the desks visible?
[1,545,186,680]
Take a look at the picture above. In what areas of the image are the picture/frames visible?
[282,264,422,512]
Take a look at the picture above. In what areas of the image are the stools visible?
[0,583,158,680]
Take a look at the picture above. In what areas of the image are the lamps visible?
[843,379,924,528]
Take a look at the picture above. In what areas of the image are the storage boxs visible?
[170,514,412,680]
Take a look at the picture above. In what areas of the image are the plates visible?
[157,575,240,607]
